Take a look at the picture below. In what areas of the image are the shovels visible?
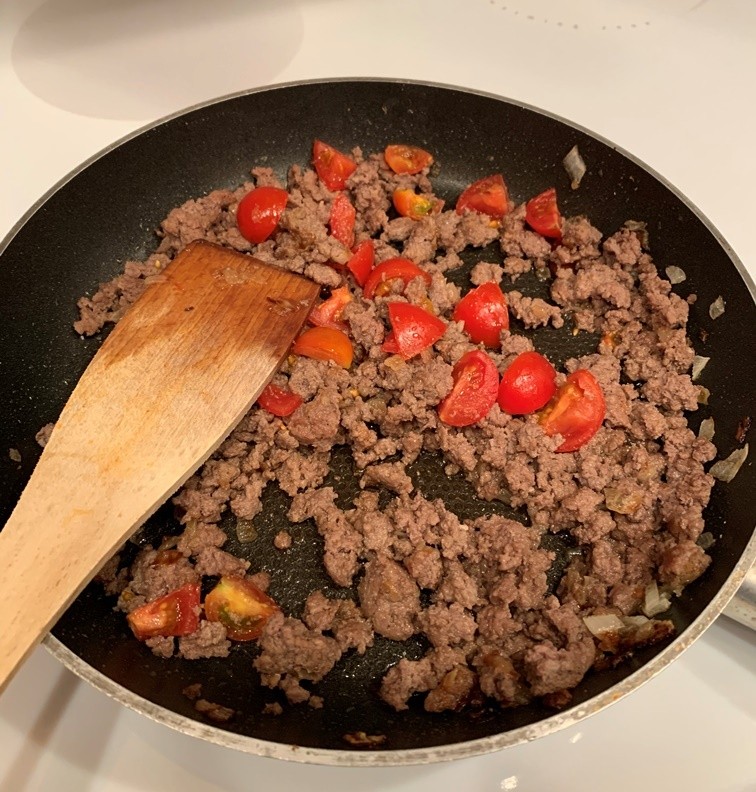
[0,237,319,696]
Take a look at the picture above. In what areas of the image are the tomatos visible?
[124,141,608,637]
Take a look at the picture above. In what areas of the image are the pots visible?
[0,75,756,764]
